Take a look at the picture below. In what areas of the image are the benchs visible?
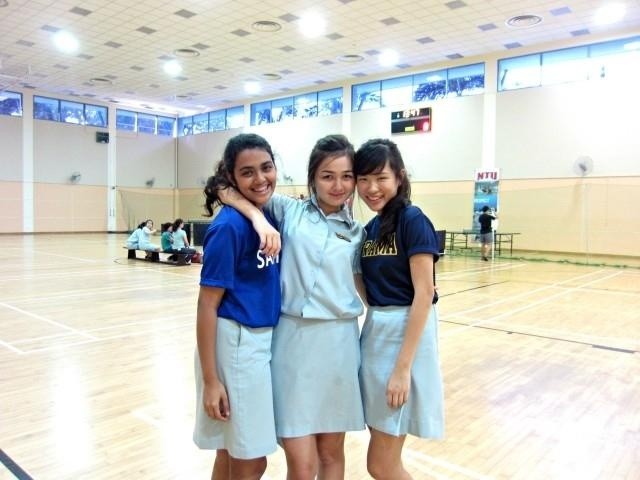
[123,246,195,267]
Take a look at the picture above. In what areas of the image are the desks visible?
[446,231,521,255]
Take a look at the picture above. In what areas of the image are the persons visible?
[479,205,499,261]
[125,218,195,265]
